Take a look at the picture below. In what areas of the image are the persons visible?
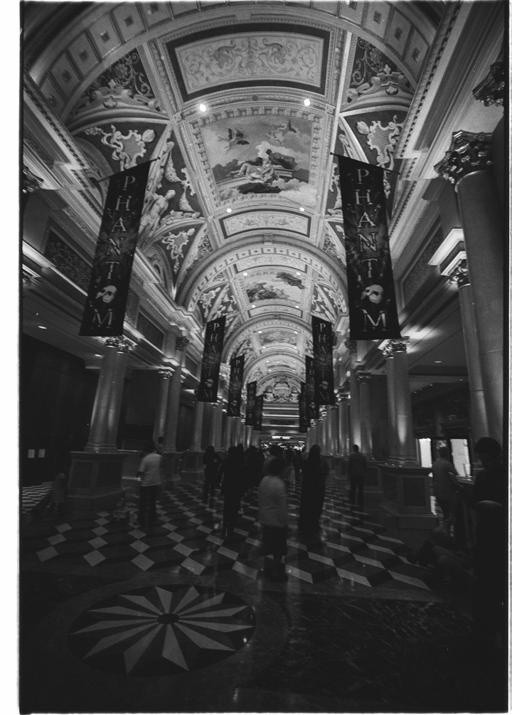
[134,435,367,585]
[433,435,508,533]
[42,435,72,510]
[427,441,459,533]
